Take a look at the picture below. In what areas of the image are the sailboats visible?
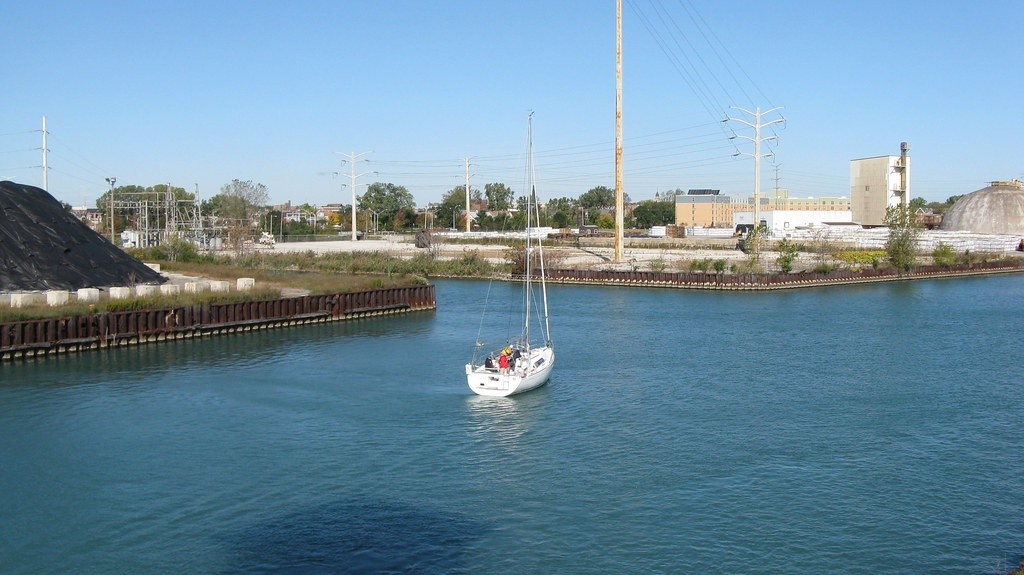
[463,110,555,397]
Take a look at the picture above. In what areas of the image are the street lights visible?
[106,177,117,244]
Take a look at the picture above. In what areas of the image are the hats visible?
[501,350,506,355]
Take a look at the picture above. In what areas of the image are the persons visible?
[485,355,498,372]
[499,347,521,368]
